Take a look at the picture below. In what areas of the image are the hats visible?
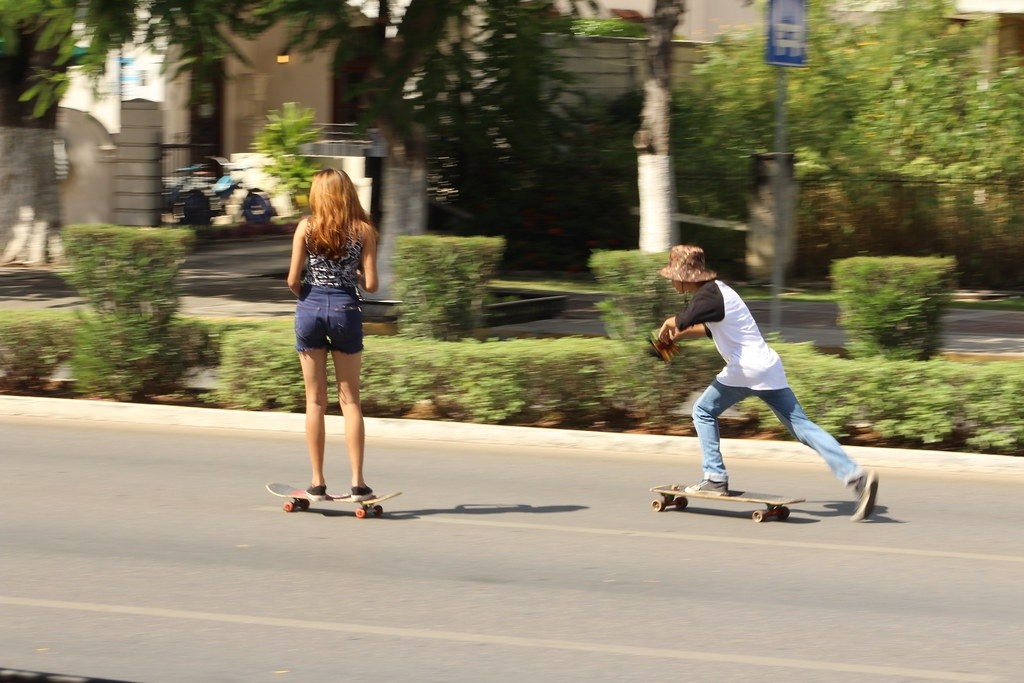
[657,245,716,283]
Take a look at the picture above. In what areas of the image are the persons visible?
[647,244,881,525]
[287,168,380,502]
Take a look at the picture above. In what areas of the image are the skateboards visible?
[265,483,404,518]
[650,482,806,523]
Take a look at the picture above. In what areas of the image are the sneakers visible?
[351,484,372,502]
[305,484,326,502]
[849,469,879,521]
[684,480,729,496]
